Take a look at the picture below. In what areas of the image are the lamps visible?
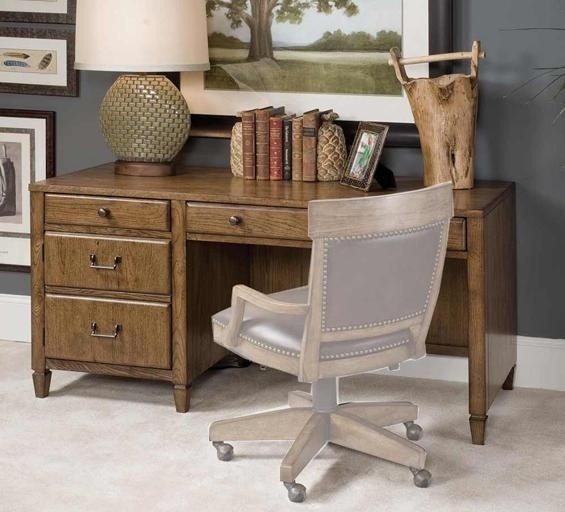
[72,0,211,178]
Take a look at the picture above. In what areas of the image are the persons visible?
[351,133,375,179]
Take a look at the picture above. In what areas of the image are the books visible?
[269,112,296,182]
[239,105,273,180]
[303,108,334,183]
[282,115,293,182]
[255,106,285,181]
[292,114,303,182]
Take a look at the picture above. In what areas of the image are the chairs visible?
[210,181,455,504]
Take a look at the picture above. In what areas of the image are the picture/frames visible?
[0,21,79,97]
[167,0,454,149]
[0,107,56,273]
[0,0,76,24]
[340,121,397,193]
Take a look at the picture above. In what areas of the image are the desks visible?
[28,160,518,447]
